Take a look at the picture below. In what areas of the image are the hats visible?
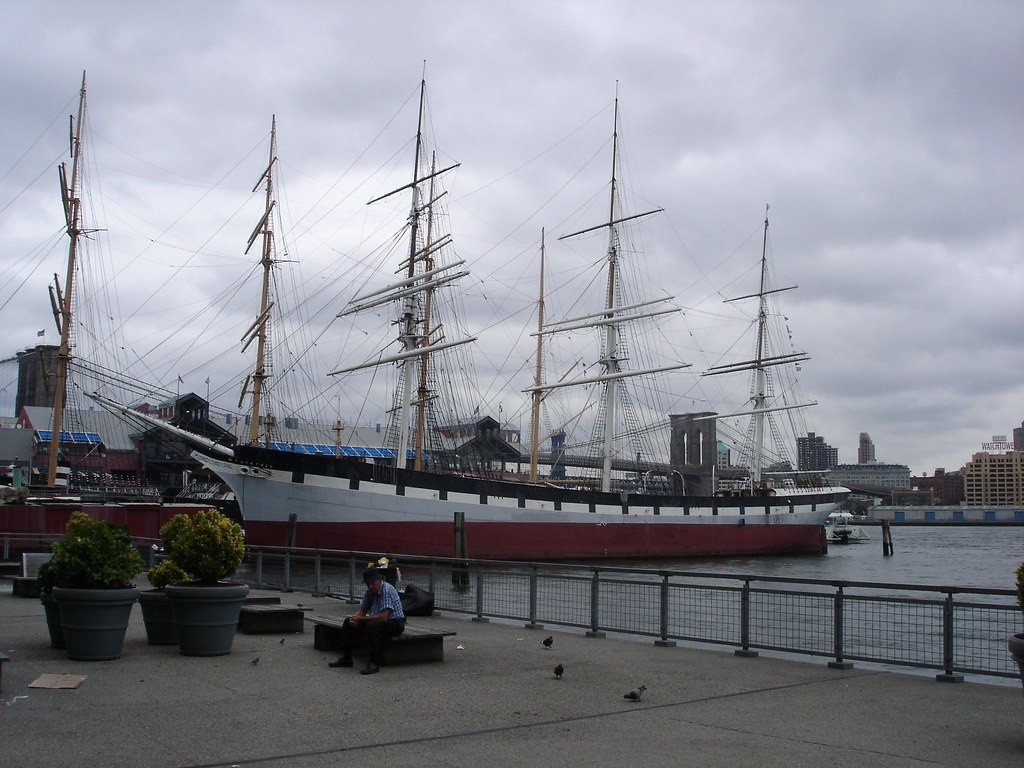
[362,568,383,584]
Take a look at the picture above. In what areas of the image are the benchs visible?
[237,596,314,636]
[304,612,458,668]
[4,552,54,599]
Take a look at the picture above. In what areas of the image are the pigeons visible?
[540,636,553,649]
[622,684,648,702]
[554,663,564,679]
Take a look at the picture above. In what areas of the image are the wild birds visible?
[278,638,285,644]
[251,657,259,665]
[296,602,304,607]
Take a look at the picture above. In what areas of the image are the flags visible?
[206,377,209,383]
[179,376,183,383]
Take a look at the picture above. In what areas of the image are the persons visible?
[329,569,405,675]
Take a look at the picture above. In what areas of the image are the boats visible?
[824,511,873,545]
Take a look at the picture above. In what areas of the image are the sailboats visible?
[0,58,855,564]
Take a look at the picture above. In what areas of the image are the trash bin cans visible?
[366,565,398,589]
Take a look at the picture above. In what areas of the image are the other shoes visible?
[361,663,379,675]
[329,657,353,668]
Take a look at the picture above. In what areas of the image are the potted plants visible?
[137,559,193,644]
[36,562,65,649]
[158,506,249,657]
[48,510,147,662]
